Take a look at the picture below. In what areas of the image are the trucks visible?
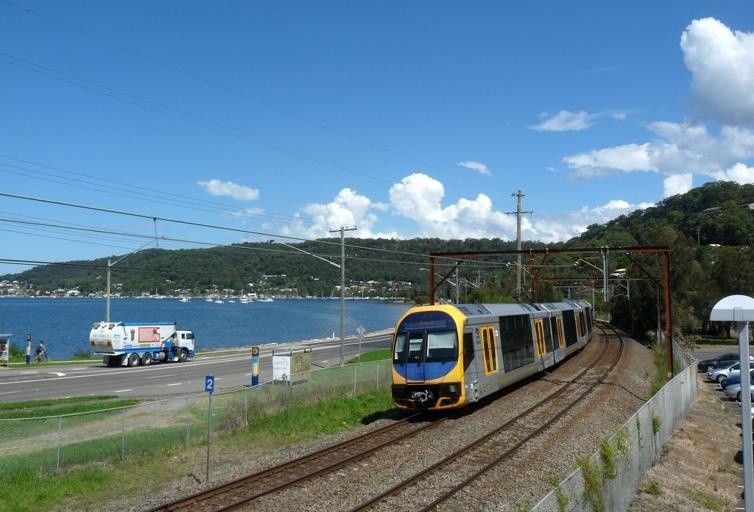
[89,322,194,368]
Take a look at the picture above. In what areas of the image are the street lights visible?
[266,238,345,367]
[105,235,168,322]
[418,268,459,305]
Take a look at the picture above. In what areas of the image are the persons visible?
[35,341,46,362]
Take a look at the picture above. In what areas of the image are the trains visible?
[388,295,594,417]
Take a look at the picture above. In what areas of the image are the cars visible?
[708,354,754,382]
[721,374,754,430]
[698,351,754,371]
